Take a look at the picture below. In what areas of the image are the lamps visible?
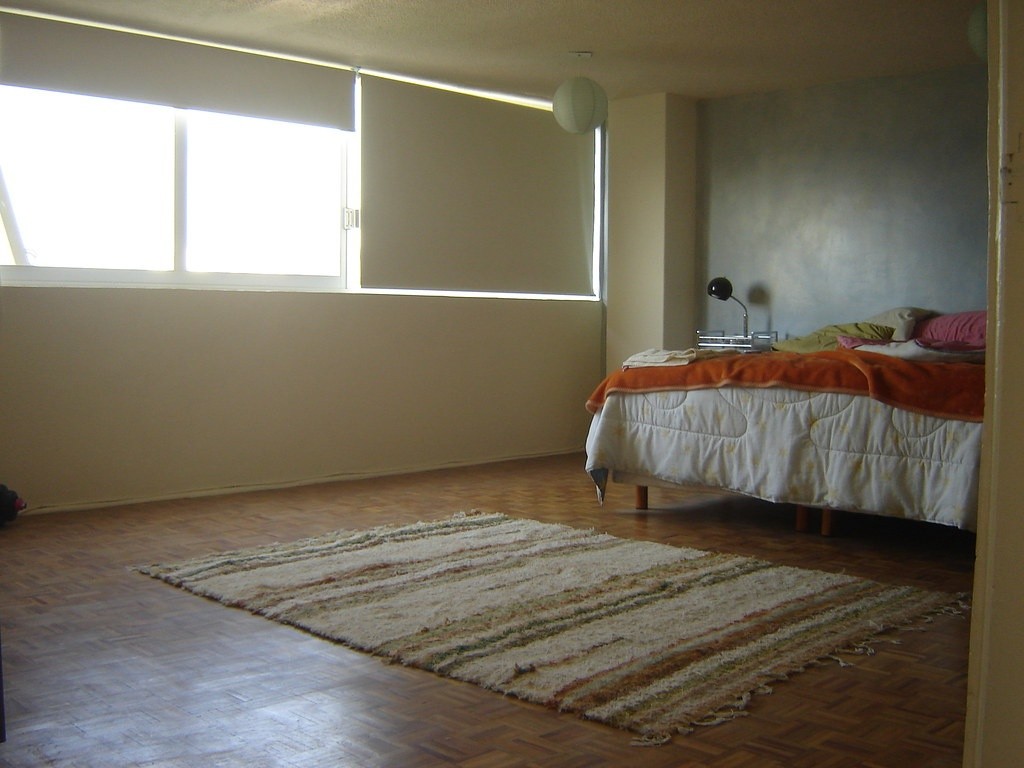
[707,276,748,338]
[552,50,609,134]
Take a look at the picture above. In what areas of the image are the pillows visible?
[775,321,899,354]
[864,306,932,342]
[914,311,985,340]
[836,334,985,363]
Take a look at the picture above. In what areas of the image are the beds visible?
[585,349,985,536]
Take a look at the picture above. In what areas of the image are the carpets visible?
[132,507,972,747]
